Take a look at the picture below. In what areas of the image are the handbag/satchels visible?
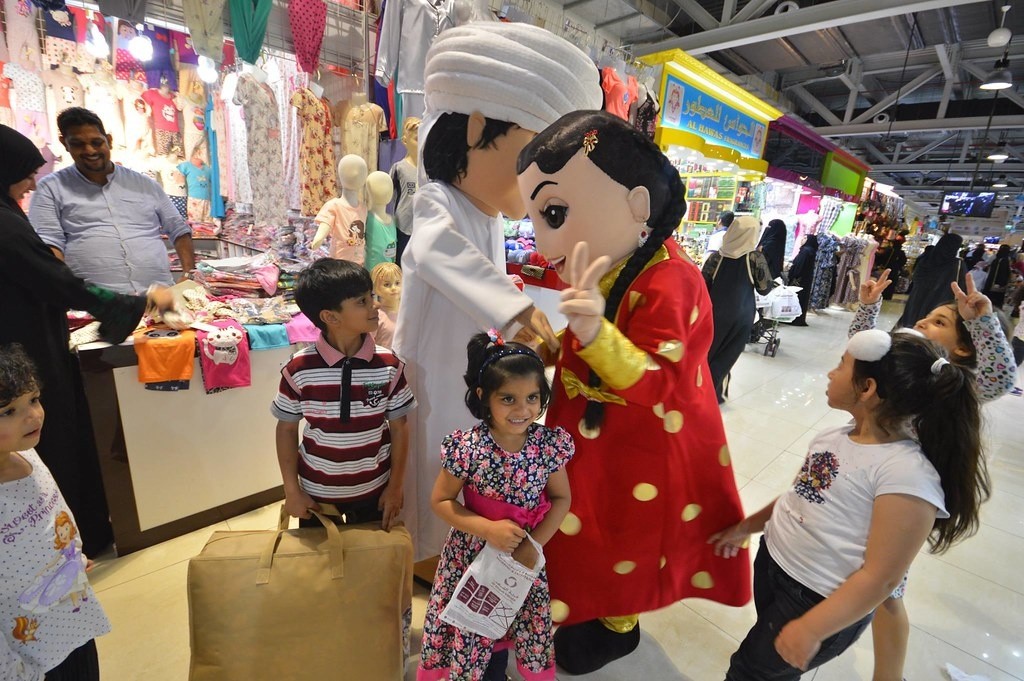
[762,285,802,323]
[438,530,547,640]
[991,285,1006,292]
[187,502,414,681]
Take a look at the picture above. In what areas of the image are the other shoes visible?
[1009,386,1023,397]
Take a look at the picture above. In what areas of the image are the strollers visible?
[745,306,782,358]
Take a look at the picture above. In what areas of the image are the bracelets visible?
[182,272,193,279]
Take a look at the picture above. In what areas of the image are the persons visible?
[779,234,818,326]
[892,233,989,329]
[27,107,195,296]
[790,209,824,259]
[382,22,603,586]
[0,342,99,681]
[272,258,418,534]
[516,111,751,674]
[311,154,367,265]
[372,116,421,350]
[417,327,575,681]
[363,171,397,270]
[983,245,1024,318]
[751,218,787,343]
[0,127,172,570]
[881,245,907,300]
[708,327,991,681]
[708,210,734,255]
[850,269,1017,681]
[702,216,773,403]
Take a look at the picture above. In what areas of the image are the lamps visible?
[986,131,1010,161]
[988,5,1012,47]
[195,49,282,102]
[993,174,1007,187]
[979,44,1014,90]
[129,23,155,62]
[85,8,110,55]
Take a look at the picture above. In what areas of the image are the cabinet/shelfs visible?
[679,175,758,223]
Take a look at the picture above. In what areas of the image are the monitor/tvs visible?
[938,191,997,218]
